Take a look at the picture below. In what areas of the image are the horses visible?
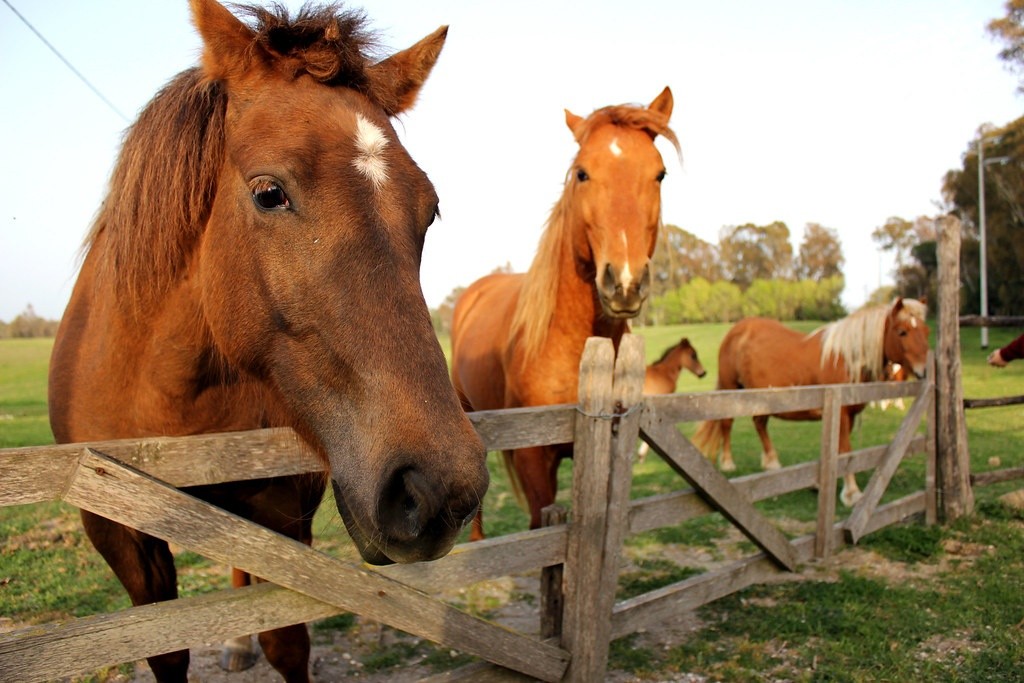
[447,80,707,545]
[690,293,933,510]
[47,0,491,683]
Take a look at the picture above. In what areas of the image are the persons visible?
[987,335,1024,368]
